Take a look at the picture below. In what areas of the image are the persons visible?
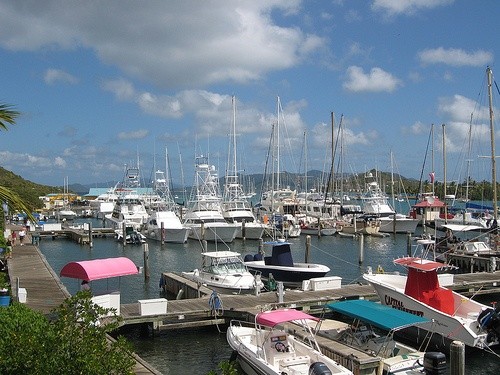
[5,216,31,259]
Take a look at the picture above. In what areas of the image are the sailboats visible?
[56,175,77,220]
[103,95,495,243]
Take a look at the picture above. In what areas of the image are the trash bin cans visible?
[18,288,27,304]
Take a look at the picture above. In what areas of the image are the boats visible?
[181,225,500,375]
